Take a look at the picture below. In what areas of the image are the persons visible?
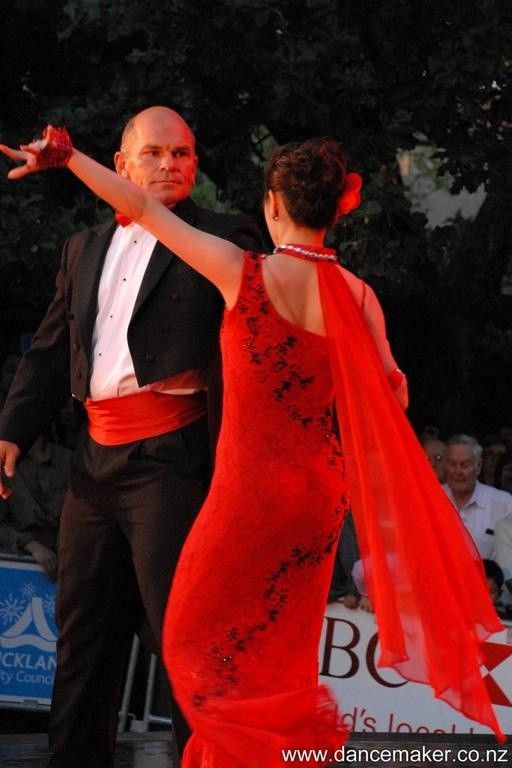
[0,103,268,766]
[0,123,510,766]
[0,422,512,624]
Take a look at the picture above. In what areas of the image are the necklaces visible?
[272,243,339,262]
